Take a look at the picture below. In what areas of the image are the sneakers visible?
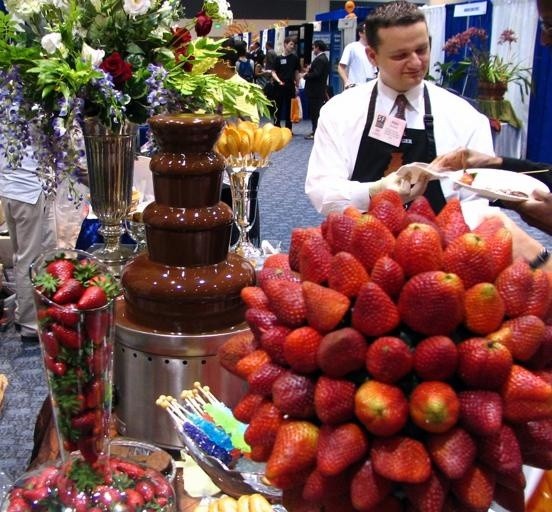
[14,322,40,343]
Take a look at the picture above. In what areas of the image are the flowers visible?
[0,0,272,210]
[442,27,537,104]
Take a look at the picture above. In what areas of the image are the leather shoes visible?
[305,133,314,139]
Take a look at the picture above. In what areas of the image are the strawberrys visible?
[8,255,173,512]
[216,189,552,512]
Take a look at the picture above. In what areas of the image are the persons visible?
[218,37,330,141]
[0,54,91,343]
[304,0,495,209]
[336,22,378,90]
[434,0,552,236]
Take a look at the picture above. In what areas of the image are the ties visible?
[393,95,407,138]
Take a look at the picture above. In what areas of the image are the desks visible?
[17,408,287,512]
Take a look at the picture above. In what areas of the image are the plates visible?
[449,169,550,202]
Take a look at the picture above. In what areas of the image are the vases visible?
[478,80,507,101]
[79,114,134,267]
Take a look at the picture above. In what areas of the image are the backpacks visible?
[238,59,253,82]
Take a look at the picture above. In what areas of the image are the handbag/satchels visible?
[291,96,303,123]
[266,81,275,96]
